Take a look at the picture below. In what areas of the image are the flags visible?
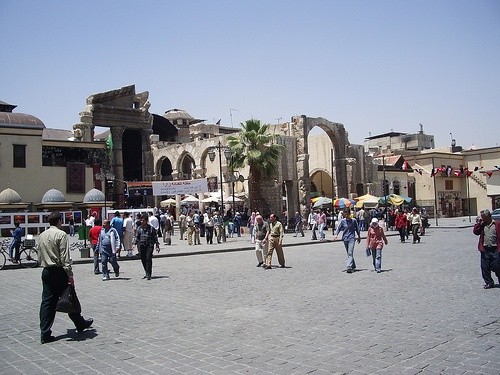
[414,164,423,175]
[402,160,408,170]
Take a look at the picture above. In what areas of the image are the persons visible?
[266,214,285,269]
[253,215,269,267]
[6,221,24,260]
[293,212,304,237]
[94,220,120,281]
[69,219,75,236]
[473,210,500,289]
[334,208,360,273]
[89,219,110,274]
[207,178,217,192]
[38,212,93,343]
[128,216,160,280]
[325,208,395,231]
[135,209,174,245]
[395,204,429,243]
[178,206,259,245]
[317,209,327,241]
[308,209,317,240]
[366,218,388,273]
[83,214,96,241]
[110,211,134,258]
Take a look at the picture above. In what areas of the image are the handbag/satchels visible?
[54,284,75,312]
[365,247,371,257]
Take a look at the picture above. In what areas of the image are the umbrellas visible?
[311,194,412,209]
[161,192,248,208]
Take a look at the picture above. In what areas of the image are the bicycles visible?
[0,237,38,271]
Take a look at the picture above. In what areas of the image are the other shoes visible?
[261,264,266,267]
[279,265,286,268]
[347,269,353,273]
[256,262,263,267]
[375,267,381,273]
[41,336,55,344]
[266,266,271,269]
[418,237,420,243]
[484,282,495,289]
[102,276,110,281]
[77,318,93,332]
[115,269,119,277]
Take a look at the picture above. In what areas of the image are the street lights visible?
[208,140,232,218]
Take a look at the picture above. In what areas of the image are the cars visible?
[491,208,500,221]
